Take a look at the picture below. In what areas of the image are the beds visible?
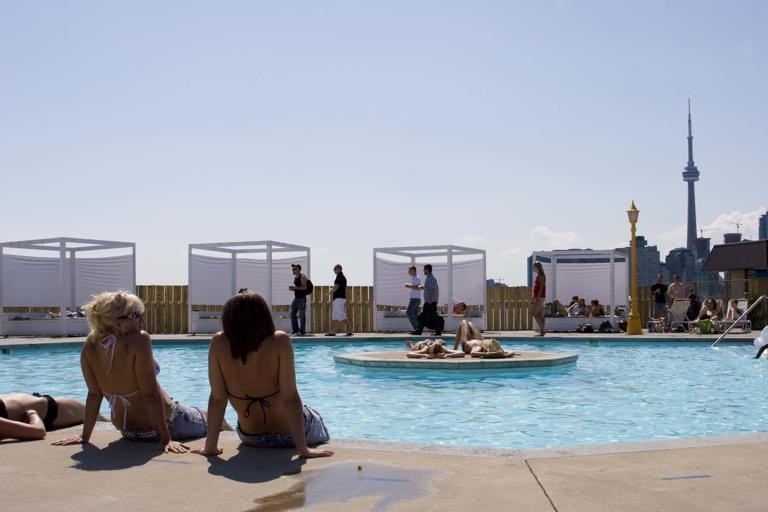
[530,247,631,331]
[372,244,487,334]
[186,239,311,337]
[1,236,140,338]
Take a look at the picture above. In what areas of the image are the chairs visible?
[647,296,752,335]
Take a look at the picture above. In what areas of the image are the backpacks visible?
[304,280,313,295]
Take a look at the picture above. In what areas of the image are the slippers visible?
[324,331,337,337]
[343,331,356,338]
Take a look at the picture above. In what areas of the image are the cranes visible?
[695,221,717,238]
[730,219,742,233]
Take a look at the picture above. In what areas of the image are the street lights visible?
[626,200,645,335]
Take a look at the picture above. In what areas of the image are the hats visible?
[291,263,301,269]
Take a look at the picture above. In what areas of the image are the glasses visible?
[115,312,141,320]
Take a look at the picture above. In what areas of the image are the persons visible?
[51,291,234,453]
[453,319,515,358]
[288,265,308,336]
[0,393,112,439]
[46,309,87,318]
[411,264,444,336]
[325,265,354,336]
[551,296,631,332]
[406,339,466,359]
[404,266,421,334]
[650,273,745,333]
[530,260,547,337]
[190,293,335,457]
